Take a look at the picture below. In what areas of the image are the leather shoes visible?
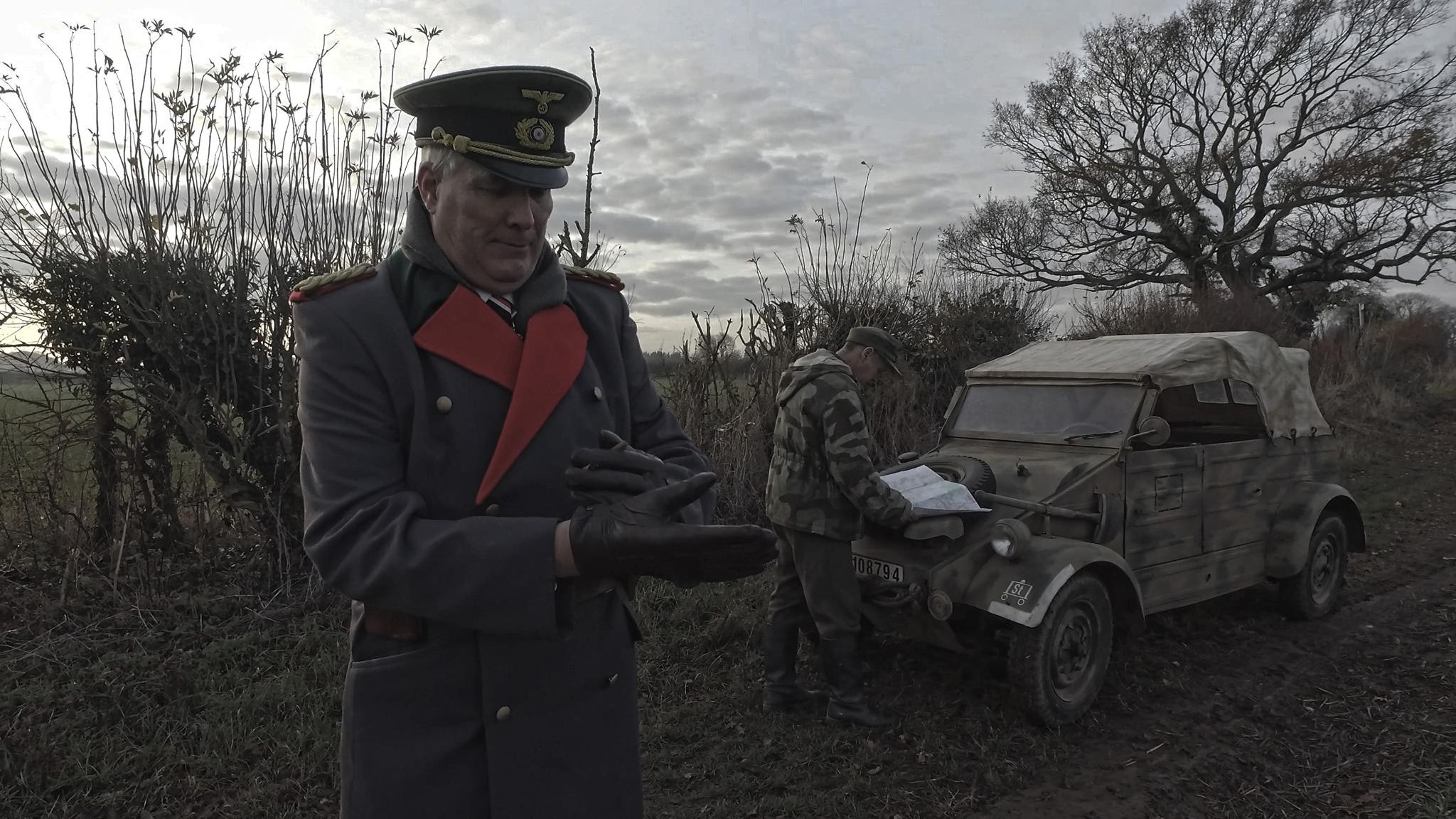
[825,696,898,727]
[762,685,825,710]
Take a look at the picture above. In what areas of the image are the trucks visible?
[853,330,1367,729]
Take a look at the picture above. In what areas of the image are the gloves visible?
[562,430,667,508]
[568,471,779,585]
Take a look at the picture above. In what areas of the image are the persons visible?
[762,325,919,730]
[288,66,777,819]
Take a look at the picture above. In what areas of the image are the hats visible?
[847,326,903,376]
[393,65,593,189]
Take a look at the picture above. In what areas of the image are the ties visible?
[487,295,526,341]
